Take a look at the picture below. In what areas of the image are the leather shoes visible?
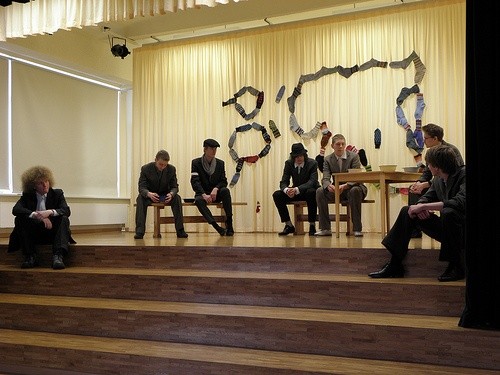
[309,226,316,235]
[279,224,295,235]
[439,262,465,282]
[369,263,403,278]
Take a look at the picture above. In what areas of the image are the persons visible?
[409,124,465,237]
[369,145,466,281]
[191,139,234,236]
[273,143,319,235]
[134,150,188,239]
[314,134,367,236]
[8,166,76,268]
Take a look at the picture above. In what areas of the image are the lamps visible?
[108,35,131,59]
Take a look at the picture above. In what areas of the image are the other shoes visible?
[217,227,225,236]
[177,231,188,237]
[53,255,64,268]
[22,256,34,267]
[134,233,143,239]
[314,230,332,236]
[410,231,423,237]
[226,228,234,236]
[353,231,362,236]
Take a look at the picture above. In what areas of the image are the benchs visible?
[134,202,248,239]
[293,200,375,236]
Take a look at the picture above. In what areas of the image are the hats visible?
[290,143,307,155]
[203,139,220,147]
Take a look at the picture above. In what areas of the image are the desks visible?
[332,170,423,239]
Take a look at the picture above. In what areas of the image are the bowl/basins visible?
[403,167,419,173]
[347,169,362,173]
[379,164,397,172]
